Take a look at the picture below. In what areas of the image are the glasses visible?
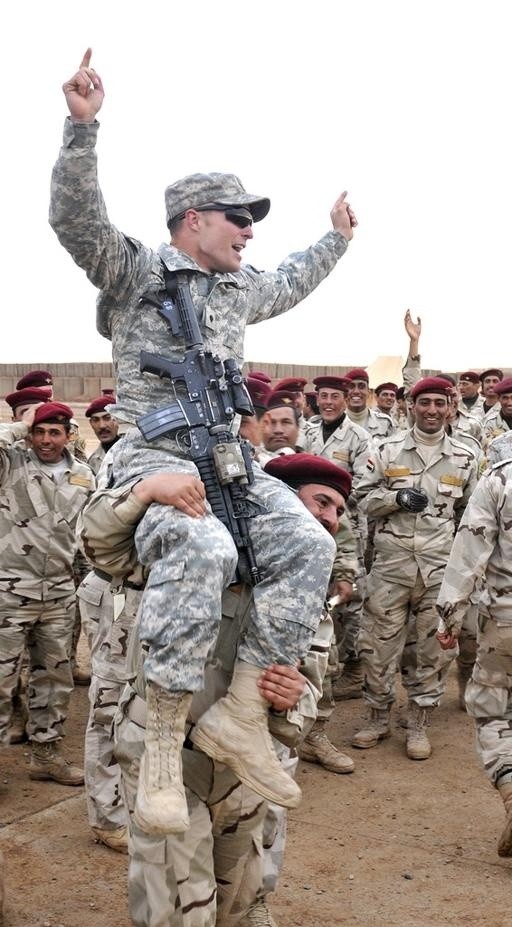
[211,208,263,235]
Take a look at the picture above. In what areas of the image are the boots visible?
[5,704,29,746]
[404,698,435,761]
[493,769,510,857]
[70,638,93,686]
[24,743,90,786]
[331,660,365,700]
[352,707,394,749]
[456,674,471,710]
[90,825,134,857]
[298,718,355,775]
[236,900,280,927]
[188,657,305,810]
[106,669,192,834]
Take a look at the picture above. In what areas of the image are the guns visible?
[132,273,263,591]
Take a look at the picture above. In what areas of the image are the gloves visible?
[395,487,429,513]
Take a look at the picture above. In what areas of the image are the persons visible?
[49,47,359,833]
[303,393,322,422]
[274,379,318,452]
[302,377,383,700]
[341,369,399,574]
[76,438,148,855]
[1,402,96,784]
[470,368,504,420]
[232,375,279,468]
[14,371,87,466]
[1,388,52,453]
[374,382,399,418]
[481,378,512,462]
[75,453,354,927]
[434,457,511,856]
[84,395,121,476]
[402,310,478,710]
[457,371,485,422]
[259,388,358,773]
[353,376,478,761]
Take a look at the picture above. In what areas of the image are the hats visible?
[478,367,504,383]
[4,388,55,409]
[312,375,353,393]
[492,377,512,396]
[161,171,276,229]
[304,391,318,398]
[247,371,272,384]
[268,389,298,407]
[373,382,399,394]
[30,401,76,429]
[459,371,480,385]
[395,387,407,401]
[411,376,454,402]
[274,377,308,393]
[344,368,370,384]
[243,378,273,406]
[263,450,354,503]
[16,369,57,391]
[83,393,117,419]
